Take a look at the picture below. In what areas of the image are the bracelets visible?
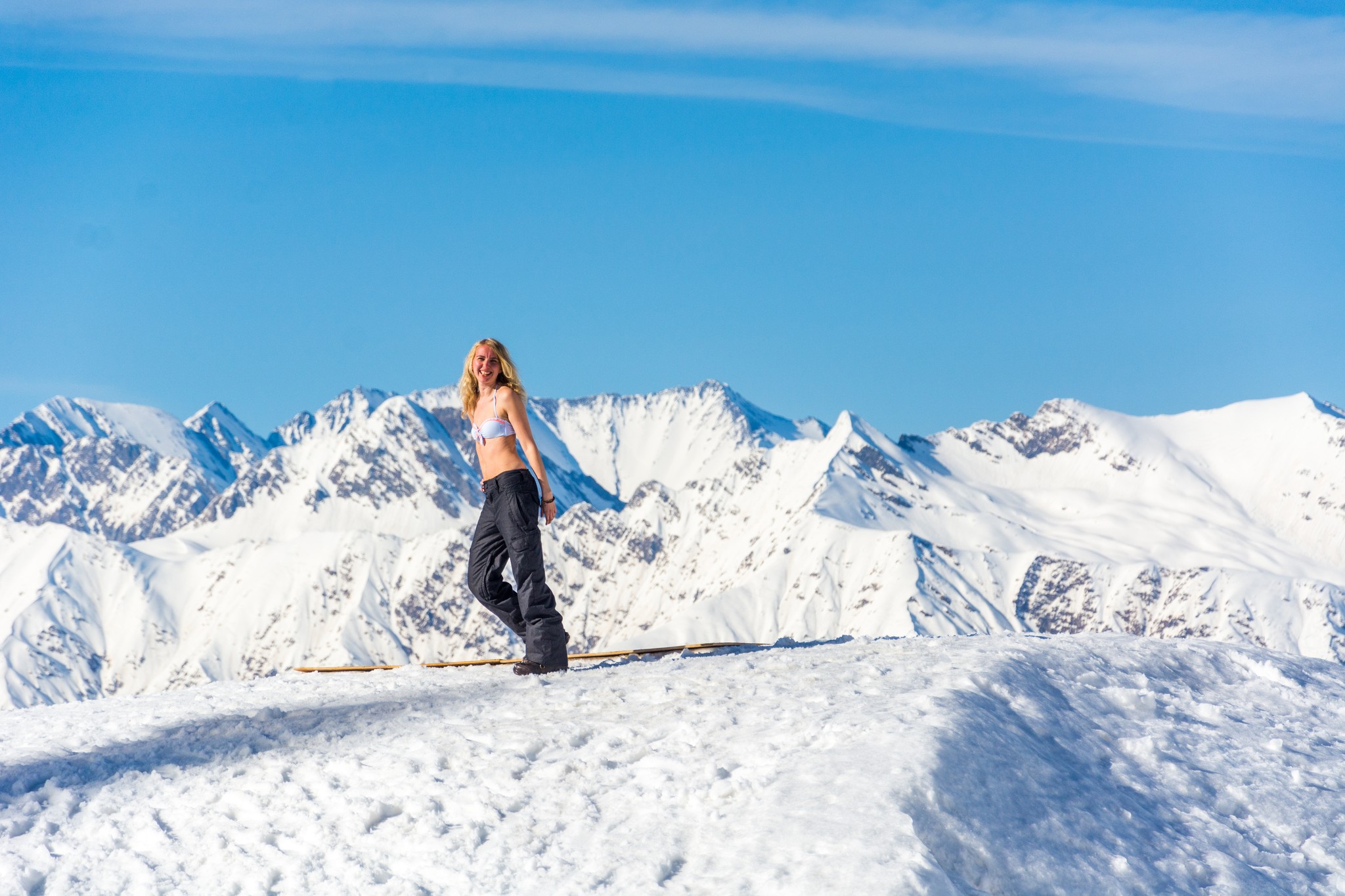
[540,495,555,507]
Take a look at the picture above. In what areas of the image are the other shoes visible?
[513,660,568,676]
[523,631,570,663]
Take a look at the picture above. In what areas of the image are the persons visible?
[457,338,569,675]
